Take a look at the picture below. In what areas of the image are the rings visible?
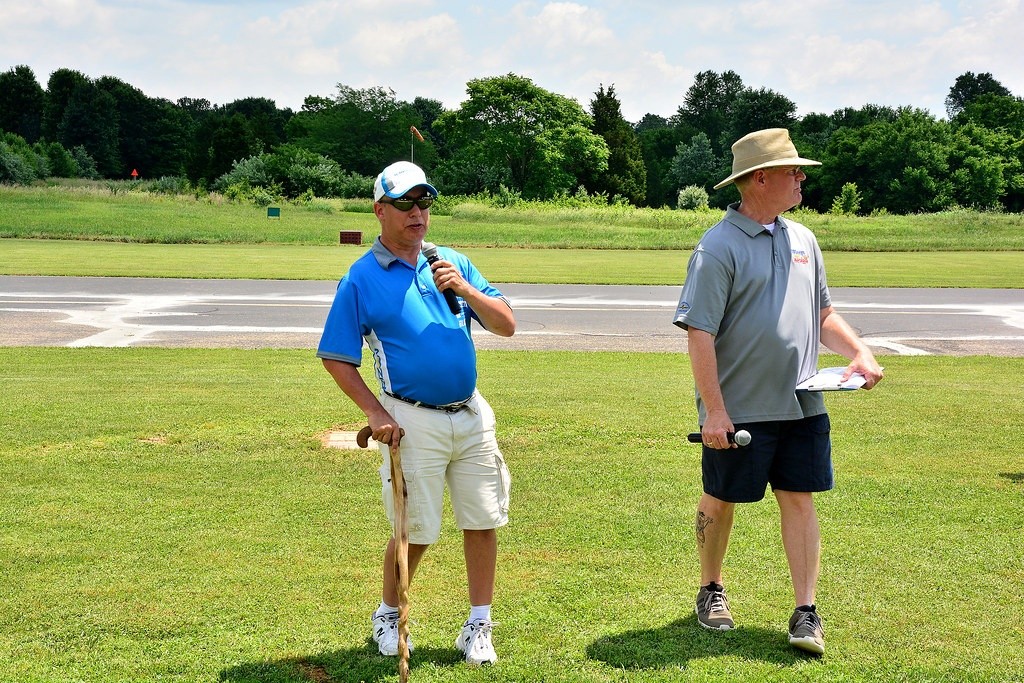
[707,442,712,446]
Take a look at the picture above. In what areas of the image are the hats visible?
[713,128,823,190]
[373,161,438,203]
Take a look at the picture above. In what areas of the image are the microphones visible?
[419,243,461,316]
[687,430,753,447]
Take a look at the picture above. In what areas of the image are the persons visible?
[673,128,883,653]
[317,161,515,668]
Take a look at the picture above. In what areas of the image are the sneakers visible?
[694,581,736,631]
[456,618,499,667]
[371,604,413,656]
[788,604,825,654]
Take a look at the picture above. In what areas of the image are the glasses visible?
[761,165,801,175]
[378,195,433,212]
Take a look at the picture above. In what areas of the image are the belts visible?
[384,390,479,416]
